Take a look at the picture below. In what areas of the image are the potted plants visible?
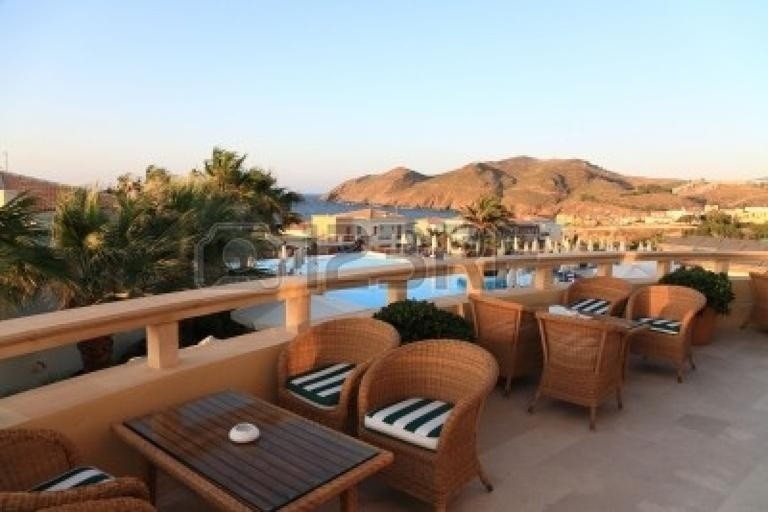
[658,264,737,346]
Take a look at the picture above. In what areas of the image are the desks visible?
[527,302,649,376]
[113,388,394,512]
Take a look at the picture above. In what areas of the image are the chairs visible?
[357,340,497,512]
[525,304,634,432]
[466,294,540,398]
[740,272,767,337]
[3,422,152,512]
[625,285,703,381]
[277,317,401,435]
[561,273,634,315]
